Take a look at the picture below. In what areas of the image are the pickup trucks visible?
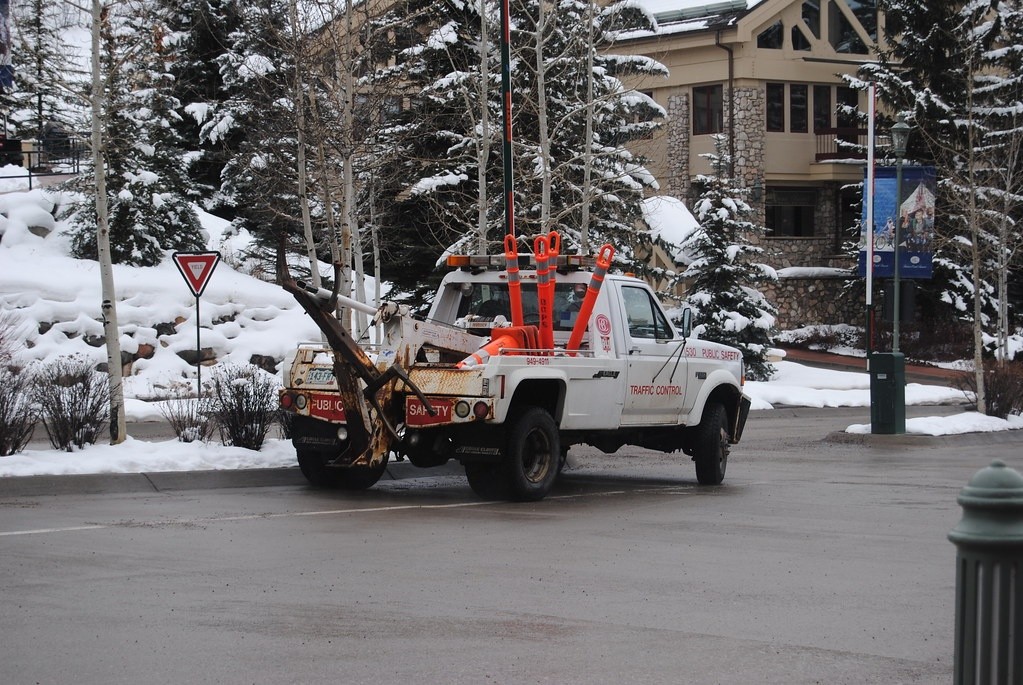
[276,254,751,503]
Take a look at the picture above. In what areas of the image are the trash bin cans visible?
[869,351,906,434]
[20,137,44,169]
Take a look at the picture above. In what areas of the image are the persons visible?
[861,207,934,250]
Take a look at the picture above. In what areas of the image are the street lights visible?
[891,112,911,352]
